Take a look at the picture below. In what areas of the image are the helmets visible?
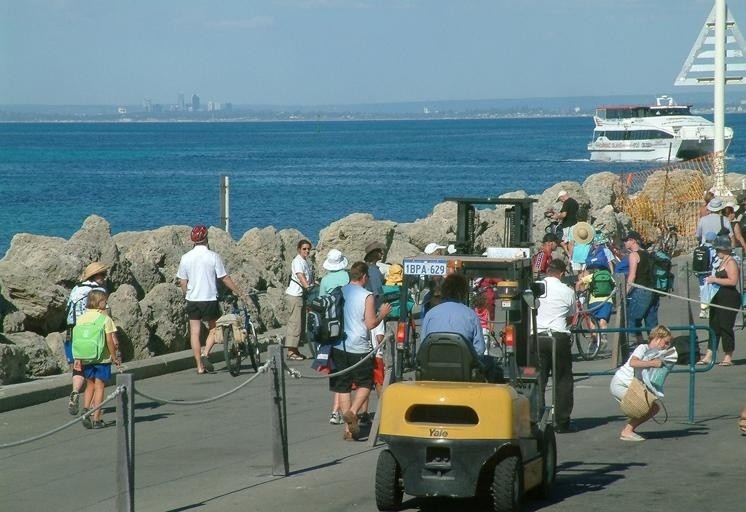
[190,224,209,244]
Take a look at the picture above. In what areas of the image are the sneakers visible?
[329,410,345,425]
[286,353,305,361]
[67,389,80,416]
[78,408,92,430]
[342,410,372,442]
[587,333,609,355]
[92,419,109,429]
[553,419,571,434]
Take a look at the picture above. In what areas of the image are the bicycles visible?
[650,224,680,257]
[572,285,603,360]
[214,288,268,375]
[394,304,418,382]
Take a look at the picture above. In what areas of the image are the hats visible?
[78,260,111,283]
[363,240,387,261]
[705,197,726,212]
[572,221,595,245]
[322,248,349,272]
[711,234,737,251]
[726,201,740,213]
[619,231,642,243]
[704,231,717,241]
[423,242,447,254]
[548,258,567,272]
[385,264,405,283]
[542,233,558,242]
[555,190,568,203]
[594,230,610,244]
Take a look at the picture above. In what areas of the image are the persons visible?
[285,192,746,440]
[175,224,250,375]
[73,290,118,430]
[64,261,111,415]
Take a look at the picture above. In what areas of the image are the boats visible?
[585,95,735,166]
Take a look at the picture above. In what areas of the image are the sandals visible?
[736,416,746,434]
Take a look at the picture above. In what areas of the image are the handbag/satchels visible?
[716,216,732,236]
[287,275,291,286]
[620,376,669,426]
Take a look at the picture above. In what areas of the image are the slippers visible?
[199,354,215,374]
[718,360,735,366]
[696,358,717,365]
[619,431,647,443]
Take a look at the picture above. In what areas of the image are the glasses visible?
[303,248,310,250]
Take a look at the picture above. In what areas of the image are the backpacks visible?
[306,285,345,346]
[648,250,675,297]
[588,269,616,297]
[691,243,713,273]
[471,276,497,309]
[71,314,107,361]
[585,245,609,271]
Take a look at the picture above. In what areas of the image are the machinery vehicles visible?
[379,192,574,511]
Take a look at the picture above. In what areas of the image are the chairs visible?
[415,332,477,382]
